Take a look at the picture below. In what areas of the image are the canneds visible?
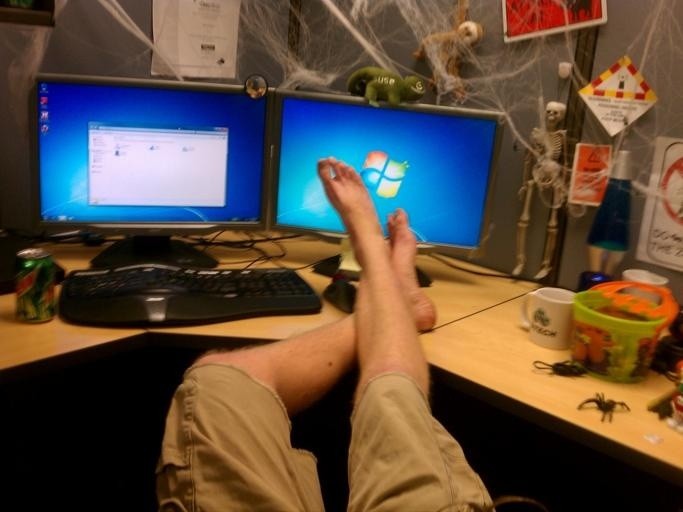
[14,248,57,324]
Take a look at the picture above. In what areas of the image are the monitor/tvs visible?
[29,71,270,269]
[268,89,507,288]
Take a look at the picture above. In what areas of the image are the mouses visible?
[322,280,358,314]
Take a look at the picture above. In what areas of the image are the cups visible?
[519,286,576,351]
[621,269,667,302]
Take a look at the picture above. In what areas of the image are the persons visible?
[157,156,496,510]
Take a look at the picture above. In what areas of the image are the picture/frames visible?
[500,0,608,44]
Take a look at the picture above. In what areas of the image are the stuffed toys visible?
[345,65,425,110]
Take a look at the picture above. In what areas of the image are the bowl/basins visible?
[569,291,671,383]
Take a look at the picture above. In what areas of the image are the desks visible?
[0,223,539,394]
[410,285,683,477]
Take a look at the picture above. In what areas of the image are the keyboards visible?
[59,262,321,329]
